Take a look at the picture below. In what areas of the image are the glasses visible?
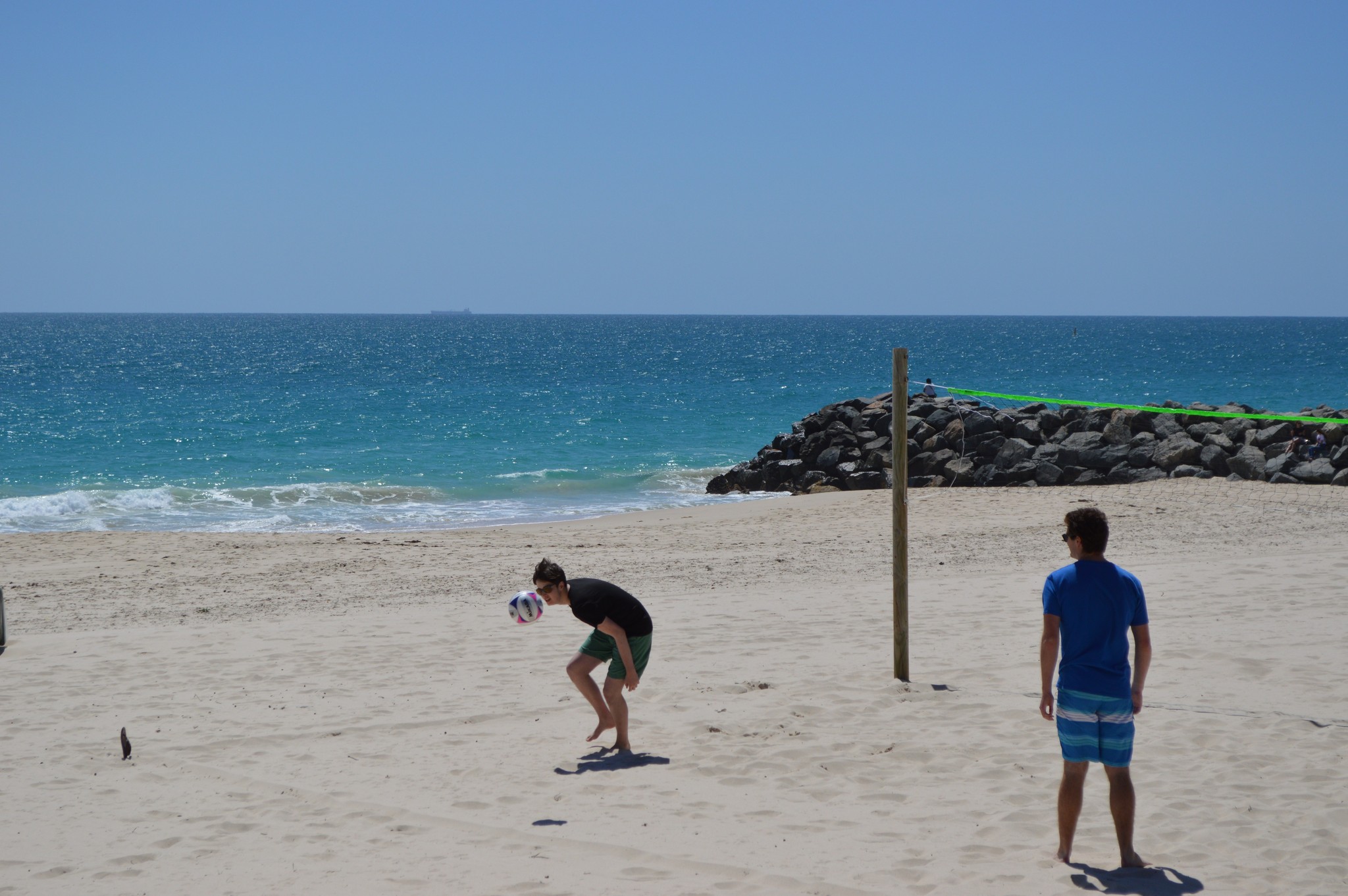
[1062,533,1076,542]
[536,582,556,595]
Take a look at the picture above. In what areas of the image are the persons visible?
[1039,508,1152,868]
[1285,420,1304,453]
[923,378,935,398]
[1308,428,1327,461]
[532,560,653,749]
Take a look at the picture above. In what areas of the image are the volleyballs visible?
[508,590,544,626]
